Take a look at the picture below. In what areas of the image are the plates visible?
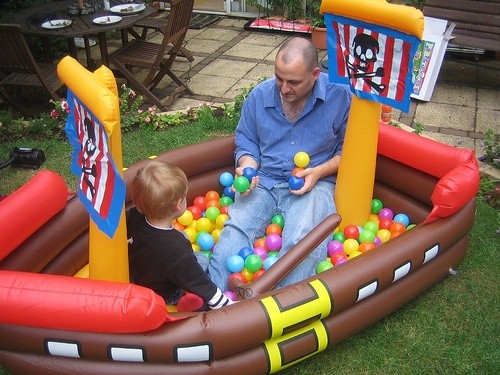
[93,15,122,25]
[110,3,146,14]
[42,19,73,29]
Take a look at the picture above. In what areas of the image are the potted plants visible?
[307,0,327,49]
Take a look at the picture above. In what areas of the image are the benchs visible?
[422,0,500,62]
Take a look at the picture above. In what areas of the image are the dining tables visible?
[17,5,158,88]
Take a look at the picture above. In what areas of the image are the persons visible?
[205,35,352,294]
[125,162,239,311]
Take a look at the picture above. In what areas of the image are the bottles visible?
[381,104,392,123]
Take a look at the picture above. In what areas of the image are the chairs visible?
[132,0,194,63]
[0,24,64,109]
[110,0,194,112]
